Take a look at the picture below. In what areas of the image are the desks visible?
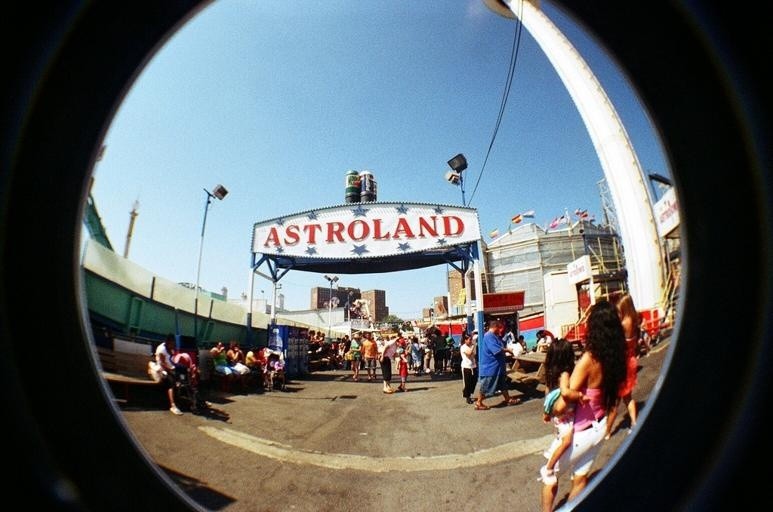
[511,351,550,390]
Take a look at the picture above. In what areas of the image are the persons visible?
[538,338,591,486]
[604,294,640,440]
[148,315,558,425]
[541,302,628,512]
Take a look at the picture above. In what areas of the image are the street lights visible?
[191,183,228,353]
[320,272,338,348]
[448,151,470,207]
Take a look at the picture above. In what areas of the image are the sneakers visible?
[539,465,557,487]
[170,405,184,416]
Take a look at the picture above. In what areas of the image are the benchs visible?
[505,370,529,385]
[96,347,167,408]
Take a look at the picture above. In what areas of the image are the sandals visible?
[474,403,490,410]
[504,398,521,405]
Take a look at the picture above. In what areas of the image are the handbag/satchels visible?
[344,351,354,360]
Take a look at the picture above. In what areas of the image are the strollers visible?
[169,355,207,415]
